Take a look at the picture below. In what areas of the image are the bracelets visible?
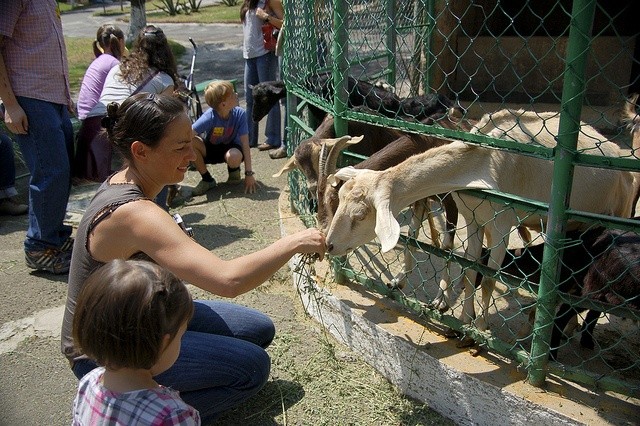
[244,171,254,177]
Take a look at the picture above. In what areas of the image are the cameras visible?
[171,212,195,239]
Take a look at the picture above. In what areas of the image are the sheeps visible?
[452,215,640,362]
[316,113,533,289]
[271,105,441,248]
[248,70,397,123]
[316,113,533,249]
[324,92,640,356]
[303,72,448,214]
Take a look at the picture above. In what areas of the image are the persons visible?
[77,24,125,124]
[0,0,76,274]
[76,24,179,183]
[269,0,335,160]
[188,80,259,201]
[240,0,284,150]
[73,259,201,426]
[61,91,326,420]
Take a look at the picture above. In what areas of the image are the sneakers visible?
[2,199,28,216]
[56,238,74,251]
[259,143,272,150]
[269,146,287,159]
[24,249,72,274]
[192,178,216,195]
[226,169,242,184]
